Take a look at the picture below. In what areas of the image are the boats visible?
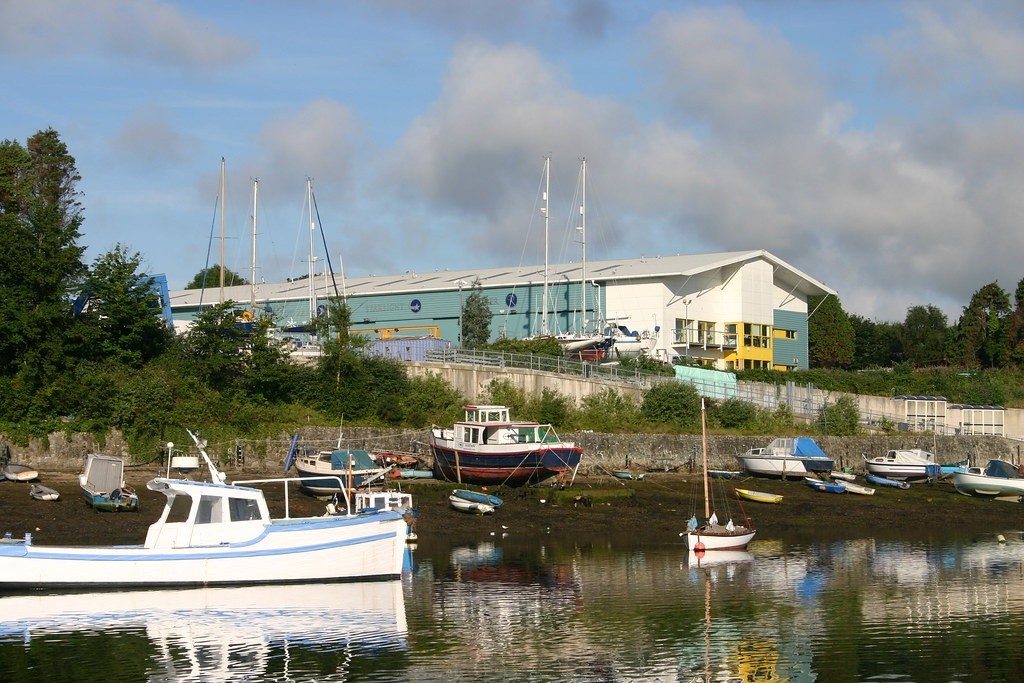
[706,469,742,478]
[865,474,910,490]
[452,487,503,508]
[834,477,876,497]
[803,476,845,494]
[678,396,757,551]
[953,458,1024,500]
[291,446,394,496]
[734,436,835,478]
[612,469,644,481]
[0,478,417,585]
[865,450,941,477]
[27,484,60,501]
[735,487,785,504]
[3,464,38,481]
[450,496,494,515]
[830,469,856,481]
[428,406,584,488]
[79,451,137,511]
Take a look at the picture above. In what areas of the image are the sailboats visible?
[495,154,663,364]
